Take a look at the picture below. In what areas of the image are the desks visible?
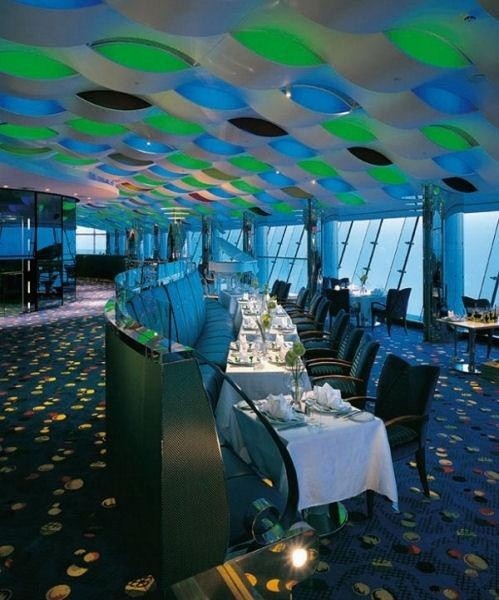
[436,316,499,372]
[232,391,398,537]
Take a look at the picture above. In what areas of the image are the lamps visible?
[161,207,191,224]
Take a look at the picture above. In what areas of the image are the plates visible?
[347,411,376,422]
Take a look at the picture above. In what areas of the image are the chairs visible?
[462,296,499,359]
[281,277,441,516]
